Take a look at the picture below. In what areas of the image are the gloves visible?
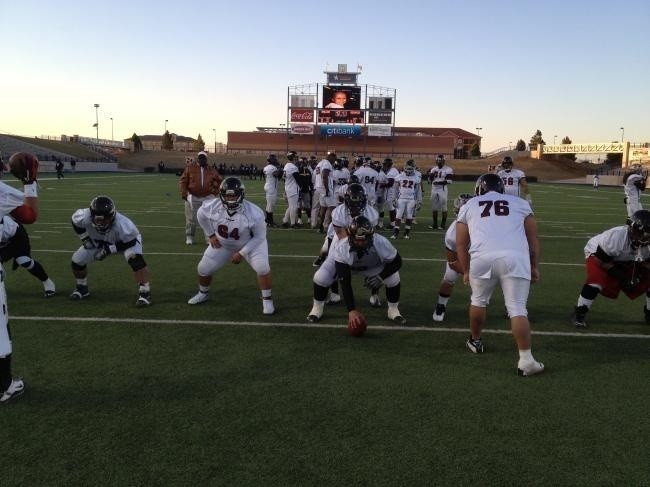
[82,238,94,250]
[93,249,109,262]
[8,151,38,184]
[363,273,384,290]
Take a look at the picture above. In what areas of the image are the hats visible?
[198,151,207,156]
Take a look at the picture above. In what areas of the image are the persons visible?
[1,161,39,406]
[456,171,545,377]
[624,164,648,220]
[70,197,153,306]
[187,177,276,314]
[213,161,263,176]
[307,183,405,329]
[263,151,425,239]
[158,160,164,171]
[433,192,473,322]
[1,216,56,297]
[428,155,454,230]
[593,174,599,189]
[38,154,118,179]
[325,92,347,109]
[572,210,650,330]
[488,156,528,198]
[179,152,222,245]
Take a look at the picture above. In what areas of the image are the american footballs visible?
[347,316,367,336]
[8,150,39,179]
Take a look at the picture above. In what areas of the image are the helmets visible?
[348,215,374,259]
[453,193,473,218]
[218,175,245,211]
[474,172,505,195]
[502,156,513,173]
[627,210,650,250]
[436,155,446,167]
[344,182,367,217]
[347,175,360,186]
[371,159,416,176]
[267,151,318,170]
[333,155,372,171]
[90,195,116,234]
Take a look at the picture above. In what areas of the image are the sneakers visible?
[312,256,325,267]
[387,307,406,324]
[572,314,590,329]
[427,226,433,230]
[185,237,193,245]
[437,226,444,229]
[432,303,447,321]
[369,295,381,307]
[187,290,209,305]
[376,221,418,240]
[306,305,324,323]
[1,375,26,406]
[135,289,152,307]
[466,336,484,353]
[265,222,325,234]
[516,358,544,377]
[42,281,56,297]
[326,292,341,305]
[263,296,275,315]
[70,284,90,299]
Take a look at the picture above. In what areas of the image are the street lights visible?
[110,117,114,140]
[93,103,100,139]
[508,141,513,150]
[212,127,217,153]
[476,127,482,134]
[554,135,558,145]
[620,126,624,142]
[163,119,169,131]
[279,123,285,130]
